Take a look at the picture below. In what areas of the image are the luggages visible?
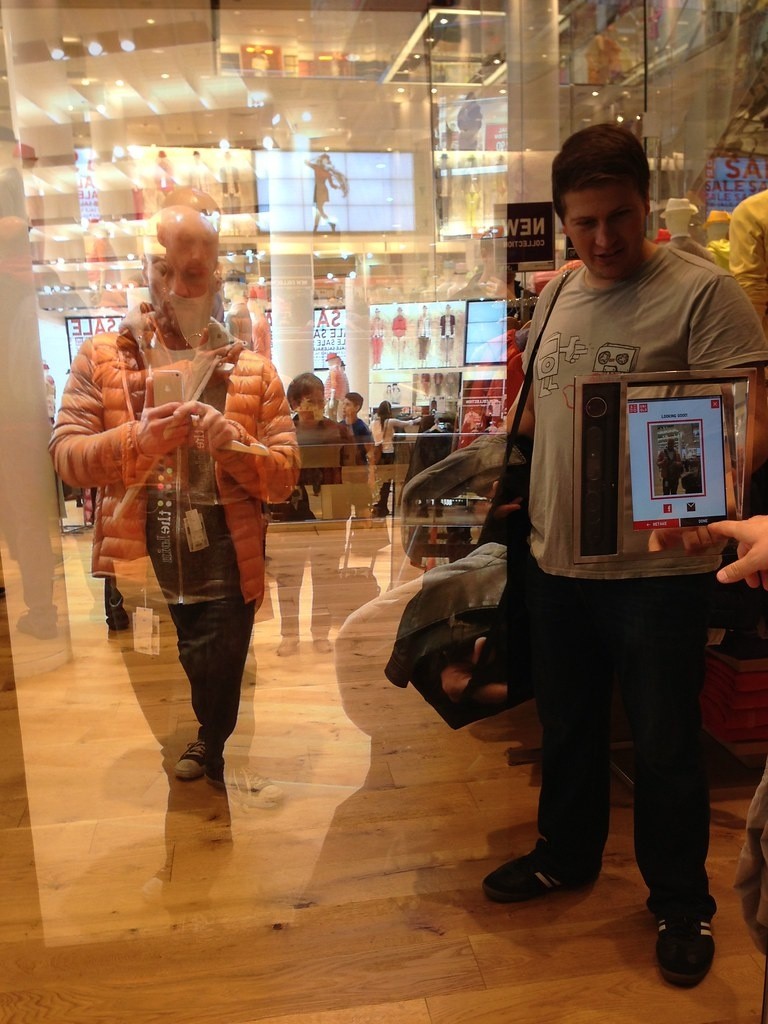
[330,516,379,623]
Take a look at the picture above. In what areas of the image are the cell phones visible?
[153,370,184,408]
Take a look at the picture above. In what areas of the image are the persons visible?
[702,210,733,274]
[658,198,714,265]
[39,92,768,990]
[707,515,768,594]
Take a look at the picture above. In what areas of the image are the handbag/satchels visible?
[373,443,383,465]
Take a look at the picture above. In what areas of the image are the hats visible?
[325,353,338,361]
[375,308,380,313]
[423,306,428,308]
[446,305,451,308]
[397,307,401,310]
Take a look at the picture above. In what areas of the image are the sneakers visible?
[481,838,600,900]
[657,912,715,986]
[172,739,214,780]
[209,763,282,809]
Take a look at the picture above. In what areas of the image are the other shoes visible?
[278,634,298,657]
[312,634,333,652]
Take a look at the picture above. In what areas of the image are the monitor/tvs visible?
[626,397,728,530]
[462,298,508,366]
